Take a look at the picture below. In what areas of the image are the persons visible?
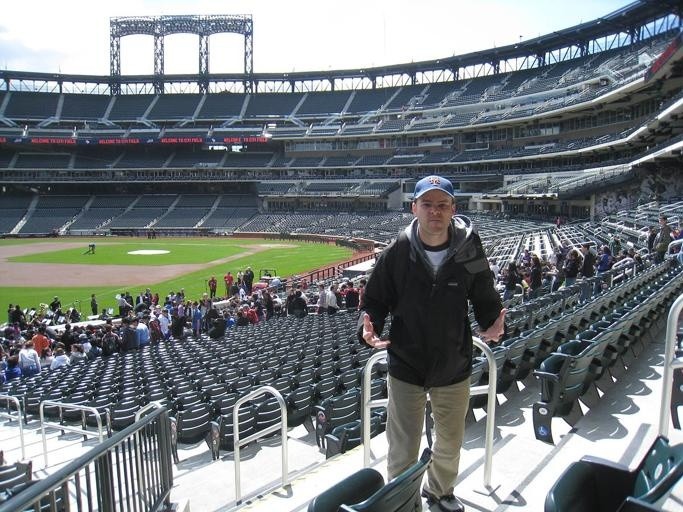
[593,168,683,219]
[88,242,95,254]
[1,267,368,384]
[489,212,682,306]
[80,229,156,240]
[356,176,509,512]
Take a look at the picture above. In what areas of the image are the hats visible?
[413,175,454,200]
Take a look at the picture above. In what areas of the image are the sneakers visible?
[421,482,465,512]
